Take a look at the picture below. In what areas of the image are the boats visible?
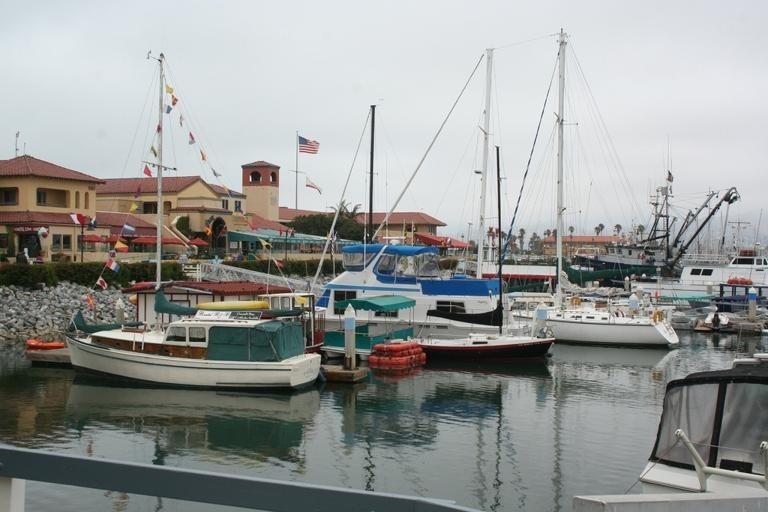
[25,340,72,362]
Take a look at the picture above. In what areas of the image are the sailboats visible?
[66,50,321,393]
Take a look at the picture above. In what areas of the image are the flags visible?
[298,135,320,155]
[305,177,321,195]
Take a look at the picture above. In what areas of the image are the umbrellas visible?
[80,234,208,258]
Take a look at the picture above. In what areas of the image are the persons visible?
[629,292,639,306]
[16,249,29,265]
